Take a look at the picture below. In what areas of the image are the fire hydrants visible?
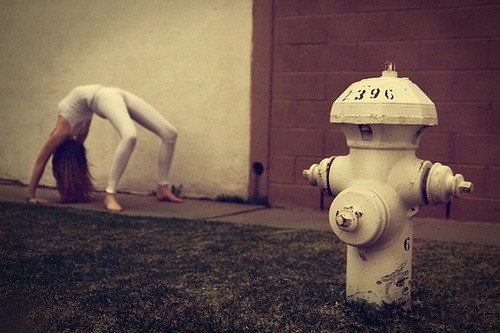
[302,60,474,311]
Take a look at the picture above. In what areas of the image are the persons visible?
[26,84,183,212]
